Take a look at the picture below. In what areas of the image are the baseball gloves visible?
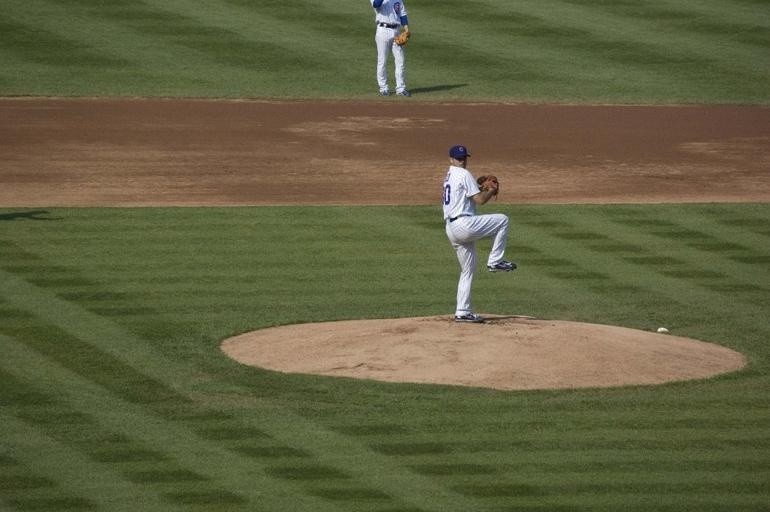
[394,31,411,45]
[477,174,498,194]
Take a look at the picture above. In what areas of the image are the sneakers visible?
[456,313,482,323]
[487,260,516,272]
[379,88,389,96]
[396,90,410,96]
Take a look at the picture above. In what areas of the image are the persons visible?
[369,0,414,96]
[441,144,518,322]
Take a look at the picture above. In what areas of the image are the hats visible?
[449,144,470,158]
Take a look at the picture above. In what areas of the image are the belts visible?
[377,21,397,28]
[450,217,457,222]
